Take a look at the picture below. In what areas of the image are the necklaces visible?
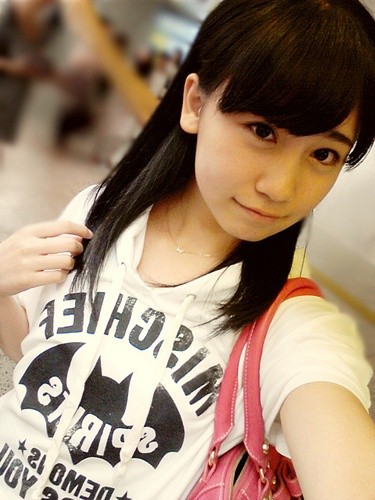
[160,200,226,259]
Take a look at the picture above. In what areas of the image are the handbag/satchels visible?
[184,277,325,500]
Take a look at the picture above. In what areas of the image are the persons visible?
[0,0,184,168]
[0,0,375,500]
